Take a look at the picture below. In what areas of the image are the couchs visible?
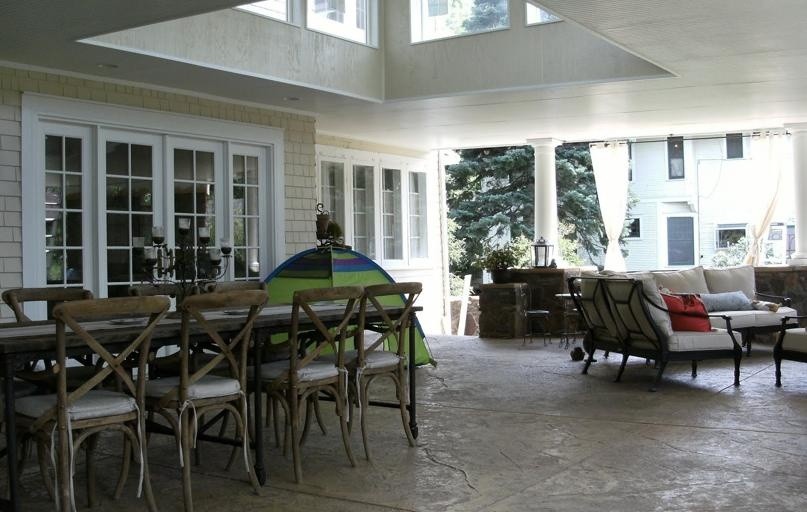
[569,277,743,392]
[583,267,799,363]
[773,316,807,387]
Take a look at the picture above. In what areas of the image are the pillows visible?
[661,293,711,333]
[700,291,754,310]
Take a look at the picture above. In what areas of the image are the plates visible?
[225,308,250,315]
[111,318,146,325]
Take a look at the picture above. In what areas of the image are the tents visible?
[250,242,437,369]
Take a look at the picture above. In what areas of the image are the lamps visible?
[143,218,232,300]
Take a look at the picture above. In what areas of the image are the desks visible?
[554,292,583,349]
[1,303,423,512]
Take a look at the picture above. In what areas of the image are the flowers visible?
[479,236,532,270]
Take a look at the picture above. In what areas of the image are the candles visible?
[220,238,230,248]
[143,246,156,259]
[179,217,191,231]
[200,227,210,238]
[208,246,220,260]
[152,226,165,238]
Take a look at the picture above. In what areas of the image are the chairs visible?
[1,361,36,501]
[133,290,270,512]
[300,282,417,461]
[520,284,552,346]
[199,281,290,438]
[225,287,364,484]
[3,289,115,448]
[129,284,204,446]
[15,296,170,512]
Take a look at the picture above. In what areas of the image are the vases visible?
[491,270,511,284]
